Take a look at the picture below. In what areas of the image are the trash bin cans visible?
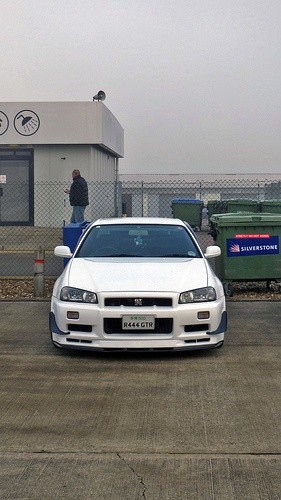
[206,198,259,237]
[258,199,281,213]
[170,198,204,232]
[209,211,281,296]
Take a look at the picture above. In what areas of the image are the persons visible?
[62,170,90,222]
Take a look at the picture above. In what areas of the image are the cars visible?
[48,217,228,356]
